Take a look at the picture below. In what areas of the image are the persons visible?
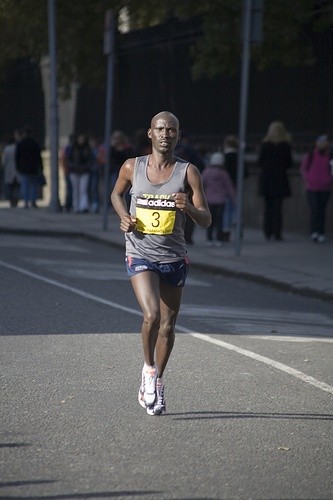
[111,112,212,417]
[0,121,333,249]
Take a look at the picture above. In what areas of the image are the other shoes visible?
[309,232,327,245]
[216,240,223,247]
[208,241,215,246]
[276,235,285,241]
[264,234,272,240]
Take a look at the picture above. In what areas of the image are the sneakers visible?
[138,364,167,416]
